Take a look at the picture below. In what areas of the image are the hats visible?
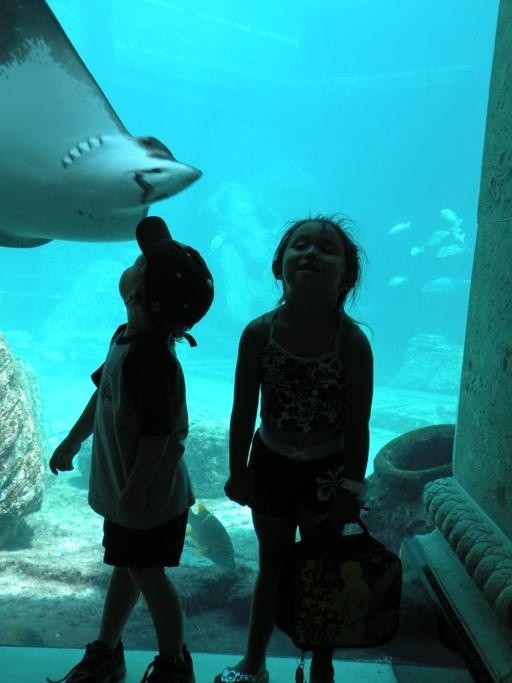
[136,215,214,346]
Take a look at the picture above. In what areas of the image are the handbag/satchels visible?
[268,519,403,652]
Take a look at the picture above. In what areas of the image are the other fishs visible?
[182,501,238,575]
[384,206,473,293]
[1,0,205,250]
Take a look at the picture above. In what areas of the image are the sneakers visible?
[144,643,196,683]
[65,639,127,682]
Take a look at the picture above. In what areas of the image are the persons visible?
[43,210,216,683]
[214,214,378,683]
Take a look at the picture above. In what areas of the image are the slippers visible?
[214,664,269,683]
